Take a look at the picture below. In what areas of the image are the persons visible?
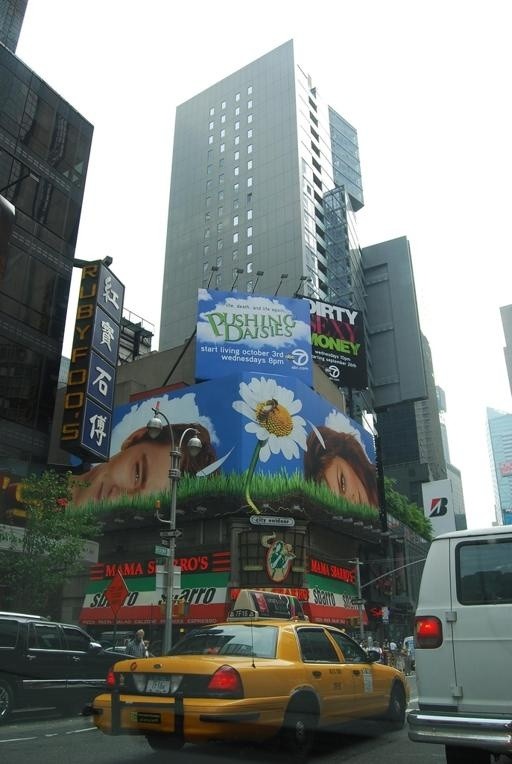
[69,418,225,503]
[125,628,147,658]
[357,635,412,676]
[143,639,151,658]
[305,422,380,508]
[208,644,222,654]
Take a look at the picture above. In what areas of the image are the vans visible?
[405,525,512,762]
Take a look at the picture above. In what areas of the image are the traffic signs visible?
[153,544,171,558]
[351,599,367,605]
[159,528,183,539]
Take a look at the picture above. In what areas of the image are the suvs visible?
[0,612,138,724]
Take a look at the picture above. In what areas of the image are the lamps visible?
[274,273,289,295]
[296,275,308,294]
[251,270,265,292]
[230,267,245,291]
[207,265,221,288]
[301,278,312,289]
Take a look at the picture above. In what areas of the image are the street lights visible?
[148,406,203,660]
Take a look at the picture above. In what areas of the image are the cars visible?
[91,618,410,757]
[103,646,156,659]
